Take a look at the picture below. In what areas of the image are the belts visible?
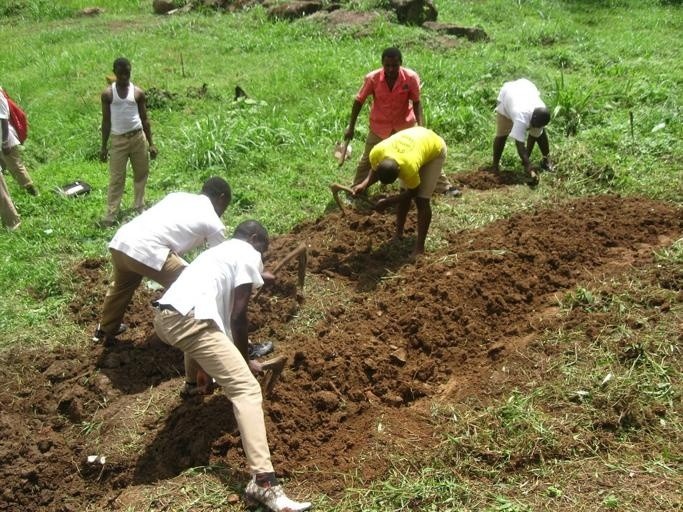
[121,128,142,138]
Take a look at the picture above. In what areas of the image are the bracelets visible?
[2,140,8,145]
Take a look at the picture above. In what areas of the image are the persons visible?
[98,56,158,227]
[348,126,447,265]
[91,175,278,360]
[0,173,23,234]
[342,45,461,206]
[152,218,314,512]
[482,77,557,181]
[0,85,38,197]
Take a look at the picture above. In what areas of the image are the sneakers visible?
[27,185,40,198]
[245,476,312,511]
[102,334,133,351]
[248,341,274,358]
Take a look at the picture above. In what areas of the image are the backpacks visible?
[1,90,28,145]
